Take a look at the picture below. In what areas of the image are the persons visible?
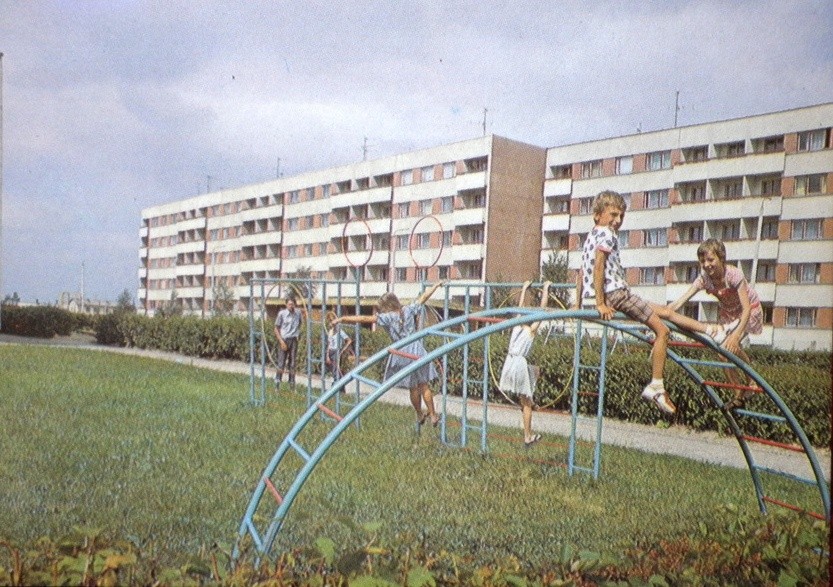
[273,298,305,392]
[326,310,351,394]
[500,280,552,445]
[664,238,765,410]
[582,190,740,414]
[330,279,449,428]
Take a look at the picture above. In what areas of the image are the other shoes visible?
[286,383,296,394]
[418,412,429,424]
[273,383,280,392]
[433,416,441,426]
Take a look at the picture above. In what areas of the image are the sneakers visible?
[642,385,676,415]
[713,318,740,345]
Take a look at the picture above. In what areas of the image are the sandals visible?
[724,399,745,410]
[744,383,760,399]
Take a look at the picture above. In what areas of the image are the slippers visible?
[521,403,538,412]
[525,433,542,446]
